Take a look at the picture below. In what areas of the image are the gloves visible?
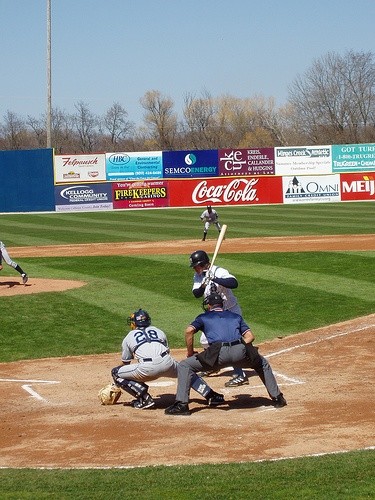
[206,270,215,280]
[202,277,210,286]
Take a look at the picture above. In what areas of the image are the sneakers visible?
[225,374,249,387]
[203,368,219,375]
[134,394,155,409]
[209,393,225,404]
[165,400,190,415]
[273,394,286,407]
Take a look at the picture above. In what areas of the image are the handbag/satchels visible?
[195,341,222,367]
[241,340,262,369]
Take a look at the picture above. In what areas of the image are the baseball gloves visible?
[98,384,122,405]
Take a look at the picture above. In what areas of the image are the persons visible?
[200,205,226,241]
[0,241,29,283]
[111,309,225,405]
[190,250,249,387]
[165,294,286,416]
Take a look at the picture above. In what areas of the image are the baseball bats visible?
[208,224,227,271]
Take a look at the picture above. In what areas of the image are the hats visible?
[207,295,222,305]
[207,205,211,209]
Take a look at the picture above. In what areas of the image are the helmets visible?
[189,250,209,268]
[127,309,151,327]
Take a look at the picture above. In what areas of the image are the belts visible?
[143,352,166,362]
[223,340,240,346]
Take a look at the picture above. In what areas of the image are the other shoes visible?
[202,238,205,241]
[22,273,28,283]
